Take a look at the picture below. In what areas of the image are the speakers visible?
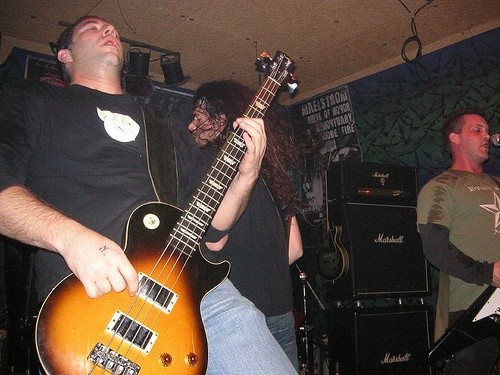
[433,336,500,375]
[304,203,432,375]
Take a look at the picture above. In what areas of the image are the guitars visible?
[428,283,500,363]
[315,152,349,281]
[35,51,299,375]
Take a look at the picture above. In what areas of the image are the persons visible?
[179,79,303,374]
[0,15,299,375]
[416,113,500,375]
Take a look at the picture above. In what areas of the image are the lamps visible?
[124,44,154,95]
[160,53,192,88]
